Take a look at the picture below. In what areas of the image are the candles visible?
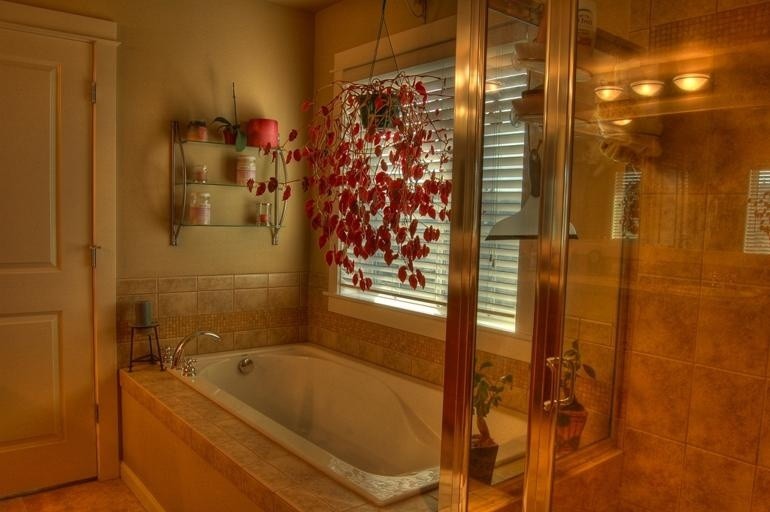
[135,300,154,335]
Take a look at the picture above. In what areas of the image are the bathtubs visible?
[157,336,527,505]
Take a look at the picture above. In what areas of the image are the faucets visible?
[171,328,223,367]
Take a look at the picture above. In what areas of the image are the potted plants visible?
[210,82,248,152]
[245,72,453,292]
[470,357,514,485]
[556,339,598,455]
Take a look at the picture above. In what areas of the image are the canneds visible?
[191,163,207,184]
[188,193,210,223]
[255,203,271,225]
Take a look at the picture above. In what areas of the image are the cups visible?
[136,300,152,329]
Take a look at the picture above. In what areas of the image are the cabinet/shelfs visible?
[168,120,290,246]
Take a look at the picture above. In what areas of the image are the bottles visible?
[189,192,213,226]
[190,165,208,184]
[254,201,273,226]
[190,120,208,142]
